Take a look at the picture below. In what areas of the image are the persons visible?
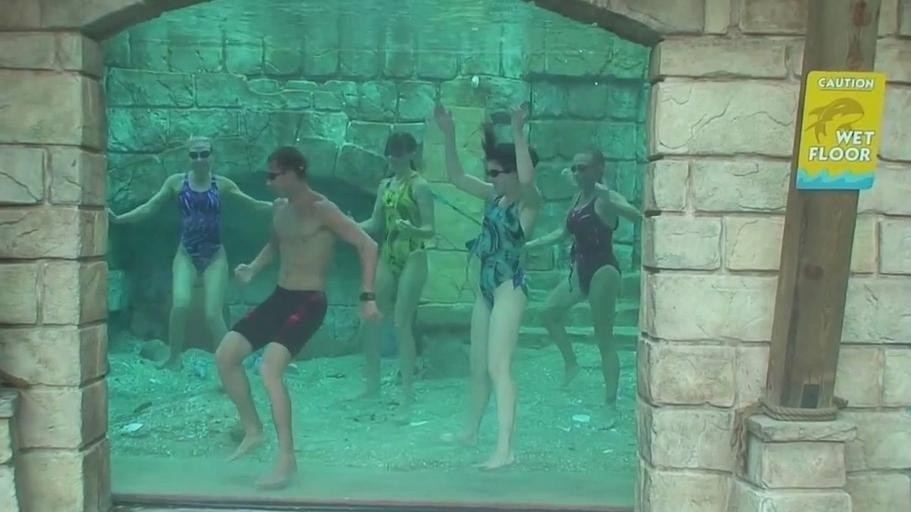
[211,146,380,491]
[521,146,644,429]
[103,133,289,391]
[348,130,434,424]
[434,95,544,470]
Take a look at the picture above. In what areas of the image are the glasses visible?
[571,164,595,172]
[485,168,509,178]
[188,150,213,160]
[269,169,289,180]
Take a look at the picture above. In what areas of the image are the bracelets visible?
[361,292,374,300]
